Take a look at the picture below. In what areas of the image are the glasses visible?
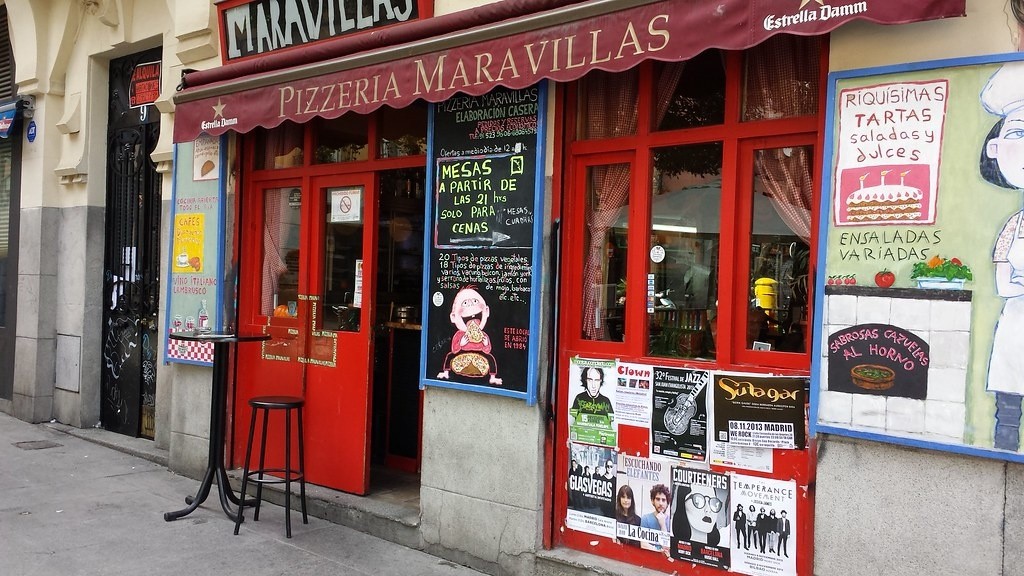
[606,465,614,468]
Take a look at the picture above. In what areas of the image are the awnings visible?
[173,0,966,144]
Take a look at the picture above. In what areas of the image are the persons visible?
[569,459,616,519]
[616,484,670,552]
[672,475,719,546]
[733,503,790,558]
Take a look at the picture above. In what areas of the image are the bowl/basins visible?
[398,306,415,318]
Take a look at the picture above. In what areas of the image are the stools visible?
[233,395,309,539]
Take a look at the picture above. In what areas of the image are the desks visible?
[164,330,273,522]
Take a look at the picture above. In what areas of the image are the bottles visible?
[414,171,421,199]
